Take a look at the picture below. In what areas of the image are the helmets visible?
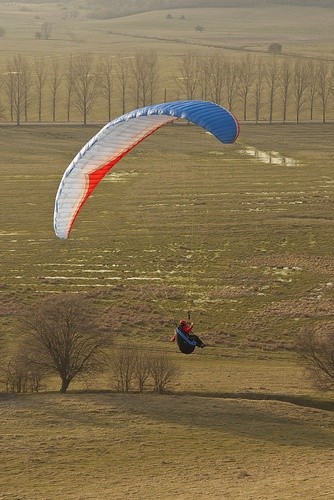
[180,320,186,324]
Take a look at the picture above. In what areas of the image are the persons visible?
[178,320,206,348]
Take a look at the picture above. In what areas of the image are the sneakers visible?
[197,343,206,348]
